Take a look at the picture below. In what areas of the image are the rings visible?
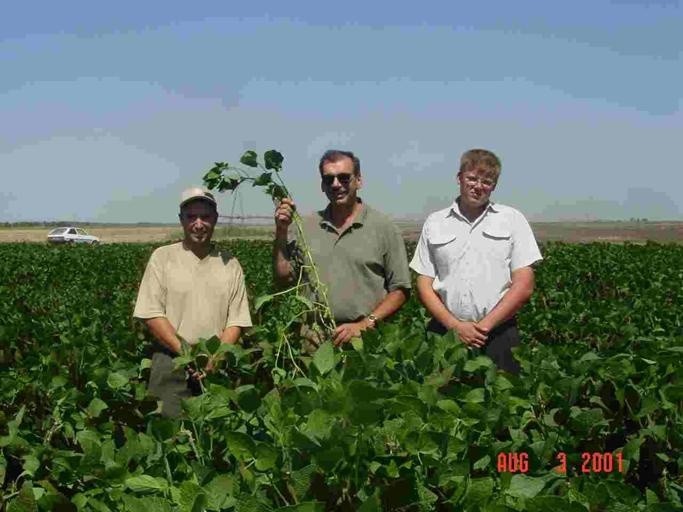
[347,334,351,338]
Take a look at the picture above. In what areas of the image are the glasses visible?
[461,174,497,187]
[322,172,355,184]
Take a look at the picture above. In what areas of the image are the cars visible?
[47,225,101,245]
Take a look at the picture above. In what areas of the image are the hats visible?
[178,187,217,210]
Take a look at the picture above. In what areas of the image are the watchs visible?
[366,314,378,329]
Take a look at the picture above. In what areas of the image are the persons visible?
[272,150,411,356]
[132,188,252,435]
[408,149,543,388]
